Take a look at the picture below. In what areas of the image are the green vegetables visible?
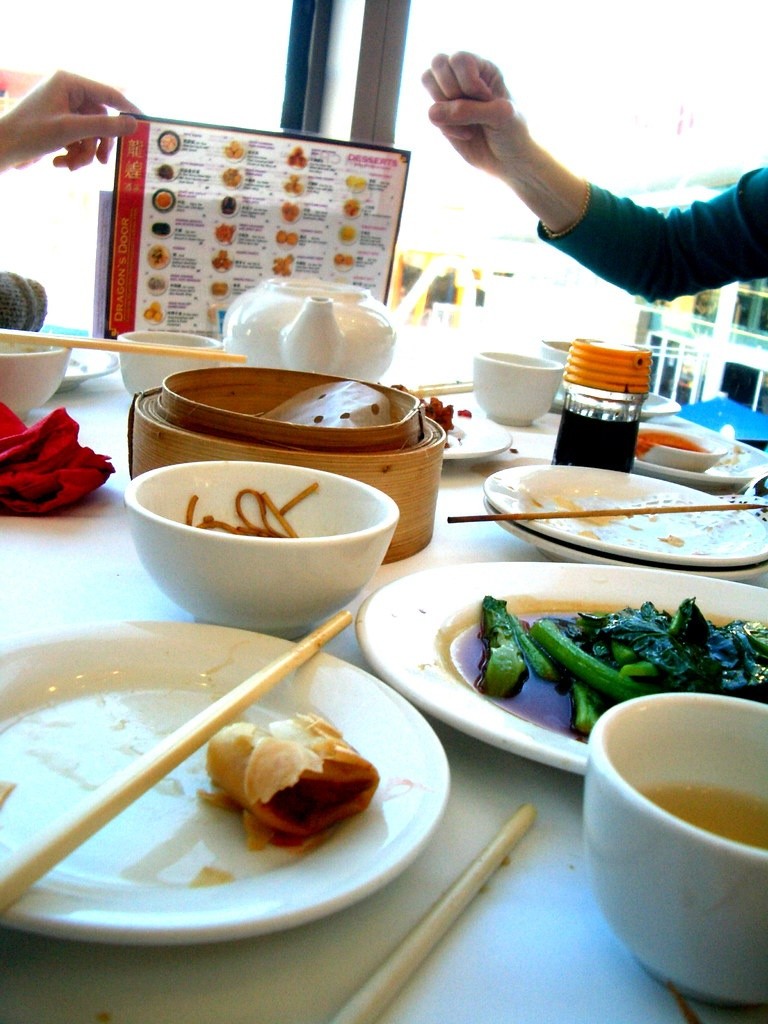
[472,596,767,735]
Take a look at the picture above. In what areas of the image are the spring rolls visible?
[205,713,381,837]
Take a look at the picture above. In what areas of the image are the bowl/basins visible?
[122,461,400,639]
[635,423,729,473]
[584,691,768,1007]
[118,329,222,396]
[0,348,73,421]
[473,352,567,427]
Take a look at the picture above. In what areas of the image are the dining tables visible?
[0,322,768,1024]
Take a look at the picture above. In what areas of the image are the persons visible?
[421,51,768,305]
[0,71,144,173]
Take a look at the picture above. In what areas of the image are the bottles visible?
[551,340,654,472]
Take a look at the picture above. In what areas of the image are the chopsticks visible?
[405,379,473,398]
[0,326,246,366]
[0,611,533,1024]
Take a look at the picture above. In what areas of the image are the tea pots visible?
[223,279,396,382]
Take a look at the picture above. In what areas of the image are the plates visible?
[483,497,768,581]
[357,562,768,773]
[56,351,117,392]
[0,621,451,946]
[556,391,681,418]
[483,465,767,566]
[634,439,768,484]
[443,410,513,459]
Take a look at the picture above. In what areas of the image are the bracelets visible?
[541,179,591,238]
